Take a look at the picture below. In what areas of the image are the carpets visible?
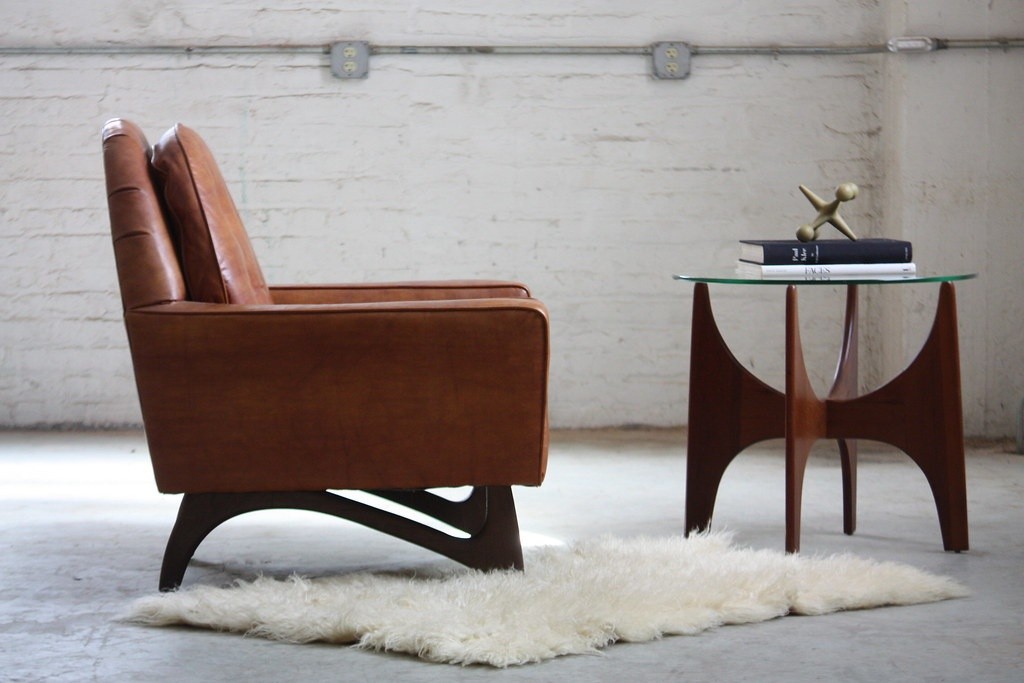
[107,520,971,666]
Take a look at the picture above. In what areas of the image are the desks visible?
[672,265,978,547]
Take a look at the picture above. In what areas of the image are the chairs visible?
[102,118,548,593]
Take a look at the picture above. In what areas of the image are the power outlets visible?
[652,41,691,80]
[331,40,369,77]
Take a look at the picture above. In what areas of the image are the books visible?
[736,239,918,276]
